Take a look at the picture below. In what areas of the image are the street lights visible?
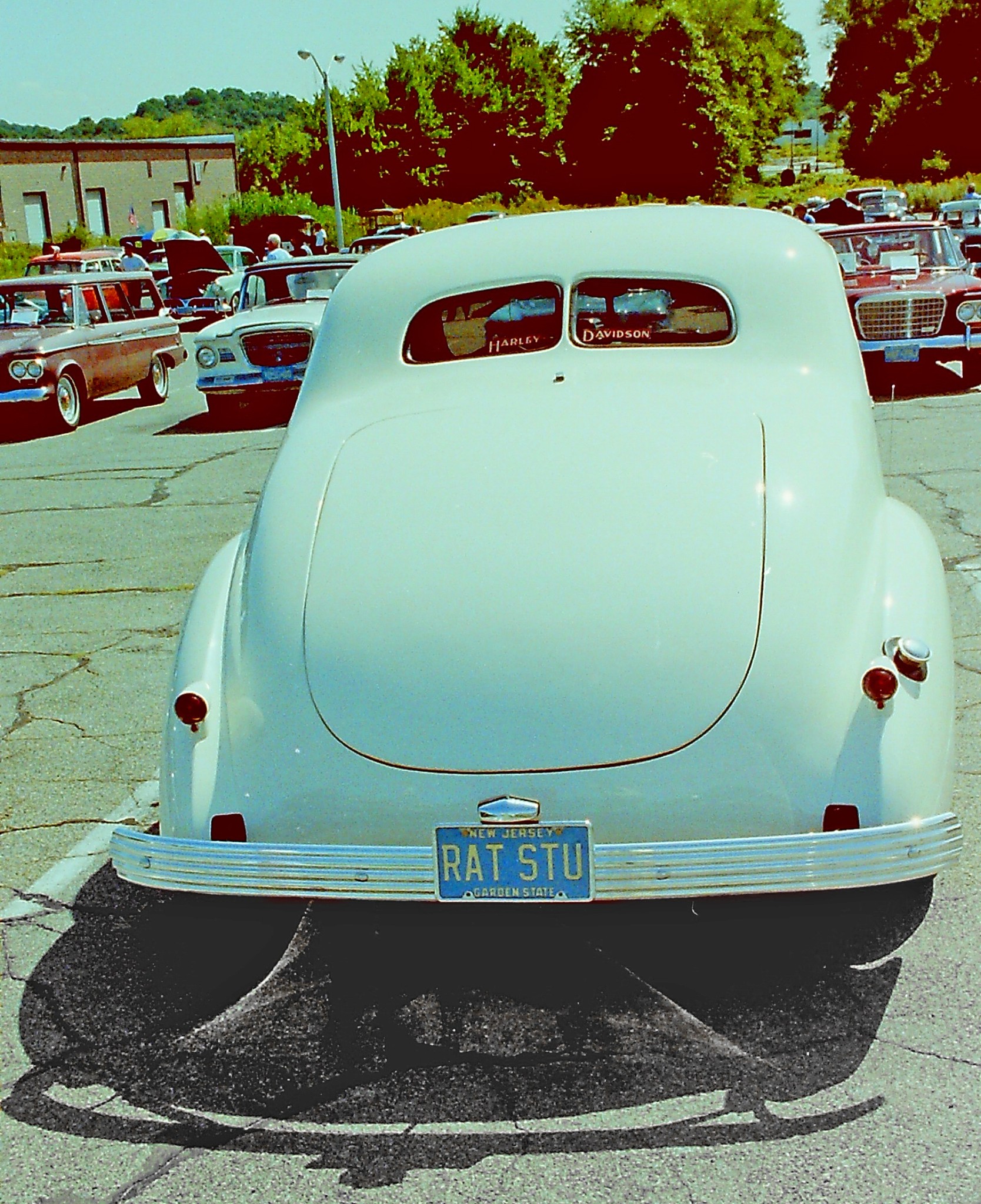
[296,47,346,253]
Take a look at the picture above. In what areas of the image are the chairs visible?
[879,248,920,268]
[836,252,856,273]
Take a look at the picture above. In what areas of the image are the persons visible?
[118,241,150,272]
[200,226,234,245]
[781,203,816,224]
[266,233,294,261]
[313,223,327,255]
[958,183,981,226]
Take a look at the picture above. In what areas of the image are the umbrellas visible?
[142,227,201,243]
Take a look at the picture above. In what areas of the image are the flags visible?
[129,204,137,227]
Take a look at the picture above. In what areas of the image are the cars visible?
[193,254,366,422]
[481,287,672,348]
[810,186,981,268]
[107,205,964,904]
[0,208,425,326]
[817,221,981,390]
[0,270,188,434]
